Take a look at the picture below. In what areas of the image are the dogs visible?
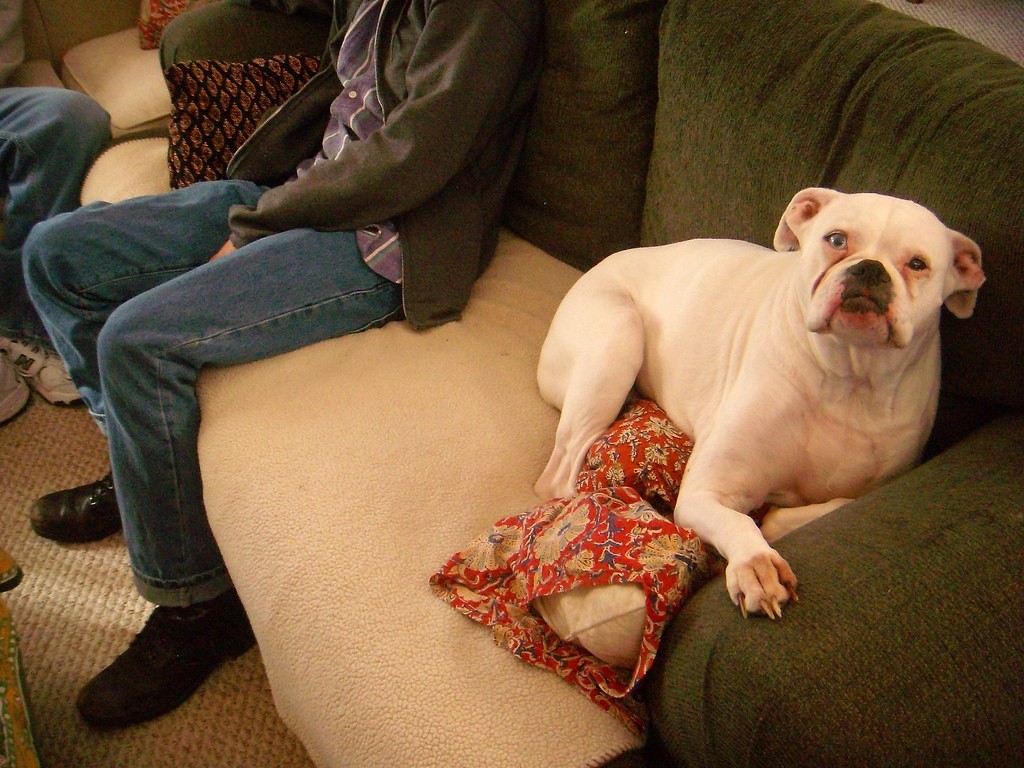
[536,188,985,618]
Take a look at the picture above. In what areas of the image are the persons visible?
[0,0,534,730]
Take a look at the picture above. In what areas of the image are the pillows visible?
[160,54,319,188]
[139,1,220,49]
[428,401,727,734]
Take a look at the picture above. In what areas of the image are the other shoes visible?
[0,547,22,592]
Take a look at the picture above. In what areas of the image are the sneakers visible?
[0,335,84,422]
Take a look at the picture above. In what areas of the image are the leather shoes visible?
[30,469,121,543]
[78,589,256,732]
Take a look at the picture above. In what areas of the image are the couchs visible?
[9,0,175,140]
[83,0,1024,768]
